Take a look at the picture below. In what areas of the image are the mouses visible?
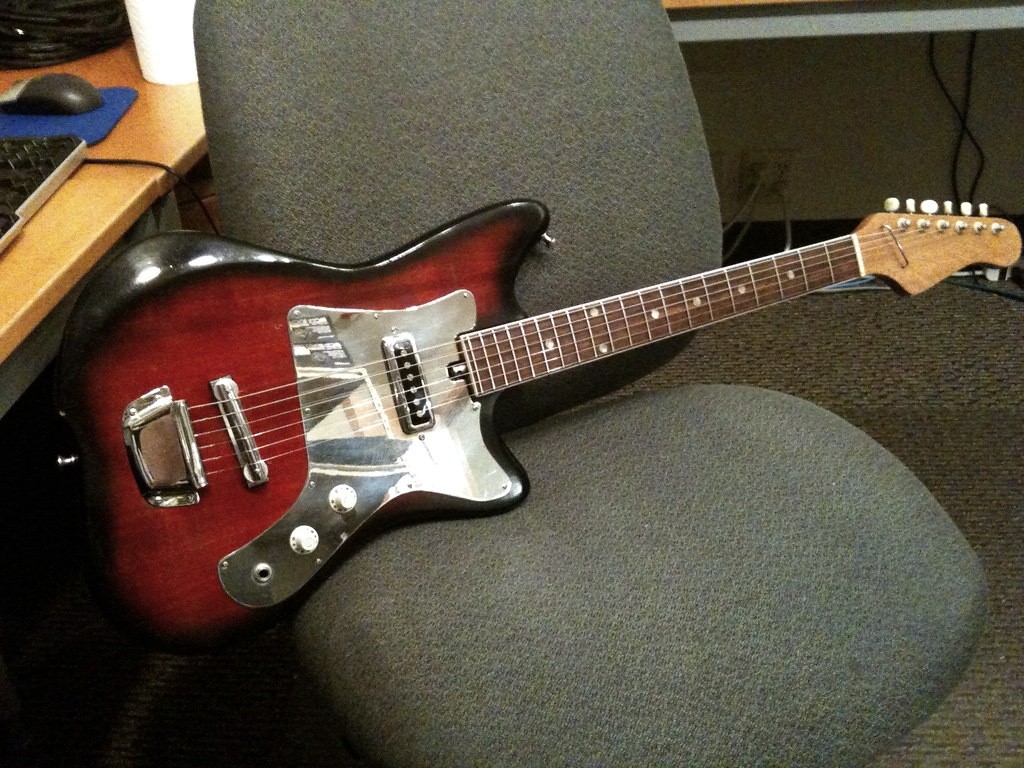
[0,72,104,116]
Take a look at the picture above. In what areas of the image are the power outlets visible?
[723,151,796,200]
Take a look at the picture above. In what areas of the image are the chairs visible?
[186,0,992,768]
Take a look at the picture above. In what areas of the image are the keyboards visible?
[0,133,87,255]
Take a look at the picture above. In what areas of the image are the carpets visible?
[0,272,1024,768]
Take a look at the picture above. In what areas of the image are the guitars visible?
[49,197,1023,651]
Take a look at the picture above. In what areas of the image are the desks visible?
[0,41,207,418]
